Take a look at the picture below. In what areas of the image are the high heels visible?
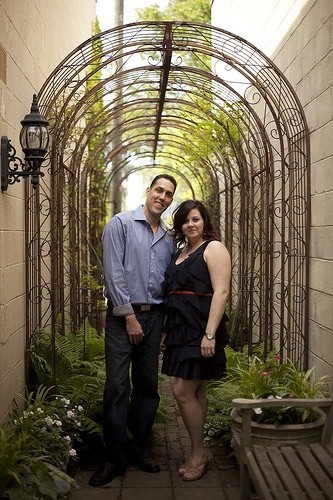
[183,449,213,482]
[178,464,190,476]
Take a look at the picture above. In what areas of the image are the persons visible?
[88,174,178,486]
[161,200,231,483]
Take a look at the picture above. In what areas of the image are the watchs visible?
[204,331,215,340]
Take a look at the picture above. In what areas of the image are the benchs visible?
[232,397,333,500]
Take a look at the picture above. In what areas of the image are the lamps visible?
[1,94,50,192]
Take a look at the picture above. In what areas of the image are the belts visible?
[107,300,163,311]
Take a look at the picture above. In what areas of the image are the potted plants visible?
[208,351,333,447]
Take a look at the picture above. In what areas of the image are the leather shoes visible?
[128,451,161,473]
[89,461,125,487]
